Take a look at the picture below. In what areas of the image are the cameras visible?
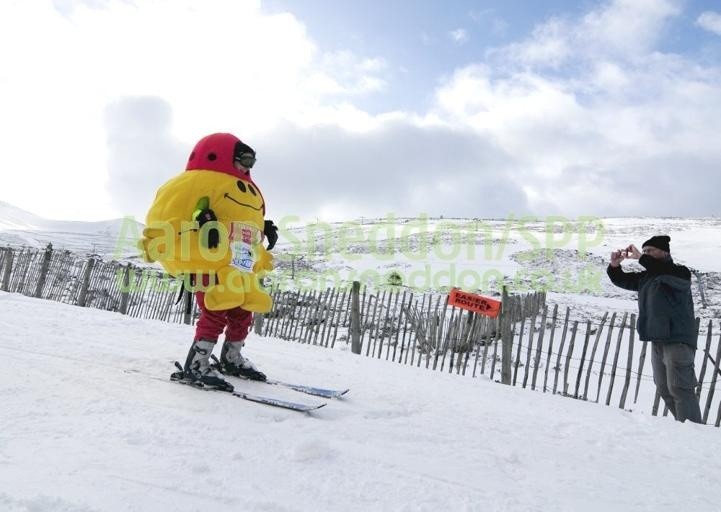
[620,249,628,258]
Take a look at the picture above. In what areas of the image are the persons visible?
[137,131,279,389]
[608,235,704,425]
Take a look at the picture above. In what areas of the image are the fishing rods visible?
[125,357,348,410]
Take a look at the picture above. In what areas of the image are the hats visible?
[643,234,670,252]
